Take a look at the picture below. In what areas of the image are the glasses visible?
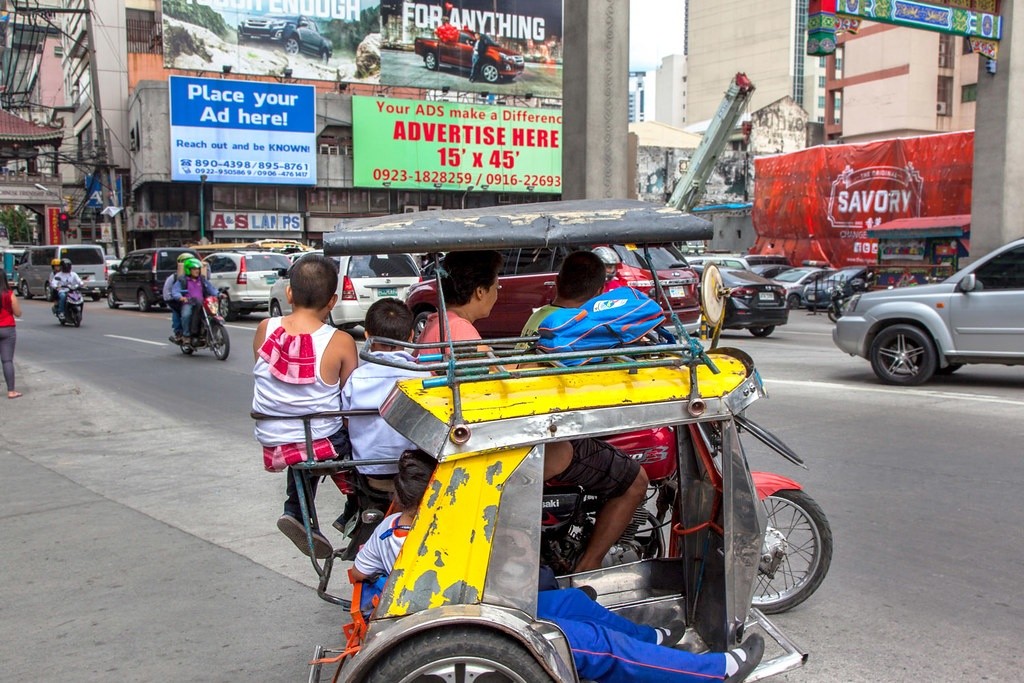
[489,280,502,290]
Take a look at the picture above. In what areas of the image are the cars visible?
[237,12,335,67]
[687,263,791,337]
[769,259,839,310]
[800,265,871,312]
[832,236,1024,387]
[679,253,796,279]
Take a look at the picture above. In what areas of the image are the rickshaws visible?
[250,197,836,682]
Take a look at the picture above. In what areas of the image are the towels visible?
[261,438,338,472]
[255,327,317,385]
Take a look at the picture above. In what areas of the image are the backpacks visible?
[534,285,666,372]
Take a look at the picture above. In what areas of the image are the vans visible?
[14,244,128,302]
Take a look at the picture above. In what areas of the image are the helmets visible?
[184,259,204,276]
[591,246,620,282]
[60,258,71,272]
[51,259,60,264]
[176,254,195,264]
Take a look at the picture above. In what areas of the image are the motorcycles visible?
[168,286,230,362]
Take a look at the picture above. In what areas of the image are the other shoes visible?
[58,312,66,320]
[332,512,355,537]
[655,620,685,647]
[8,391,22,398]
[720,632,765,683]
[181,336,191,347]
[276,511,334,560]
[53,304,58,310]
[168,335,182,346]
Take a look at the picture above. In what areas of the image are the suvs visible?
[189,239,317,324]
[106,246,203,313]
[404,242,703,340]
[268,249,424,331]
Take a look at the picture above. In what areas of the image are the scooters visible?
[50,275,89,328]
[827,272,881,324]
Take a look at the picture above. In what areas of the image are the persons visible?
[163,253,227,345]
[351,448,765,682]
[253,256,358,560]
[514,249,649,574]
[413,251,573,482]
[49,258,85,321]
[341,297,434,494]
[466,33,485,82]
[0,268,23,398]
[591,246,627,294]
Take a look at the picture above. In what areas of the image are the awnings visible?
[866,215,970,239]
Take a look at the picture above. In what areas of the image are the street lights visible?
[34,183,67,245]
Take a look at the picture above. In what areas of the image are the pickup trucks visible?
[414,30,525,85]
